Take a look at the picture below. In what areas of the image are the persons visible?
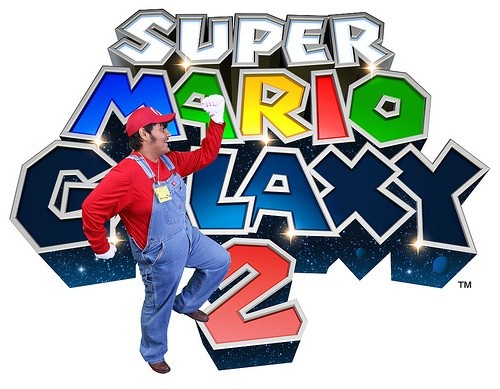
[82,93,231,374]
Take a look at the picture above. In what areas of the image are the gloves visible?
[201,94,225,123]
[95,242,117,259]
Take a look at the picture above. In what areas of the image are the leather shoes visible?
[184,310,209,322]
[149,361,170,374]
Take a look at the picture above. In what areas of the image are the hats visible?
[125,106,176,137]
